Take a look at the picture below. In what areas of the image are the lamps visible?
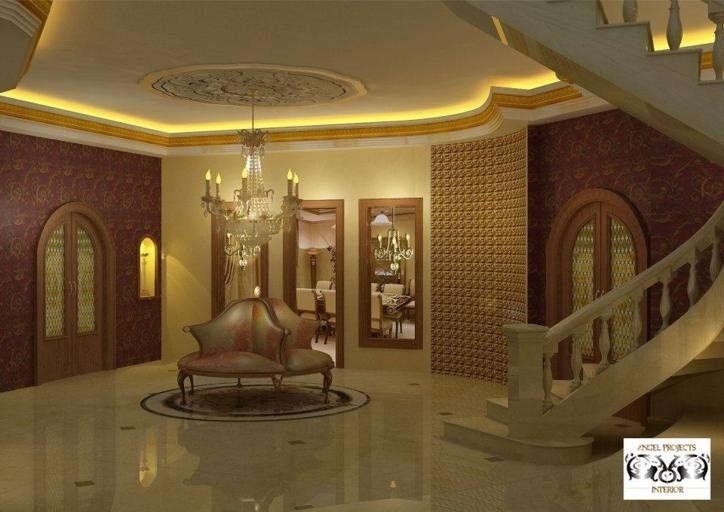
[371,205,413,276]
[0,2,52,91]
[198,81,305,273]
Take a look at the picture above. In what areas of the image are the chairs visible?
[295,278,415,346]
[176,297,334,410]
